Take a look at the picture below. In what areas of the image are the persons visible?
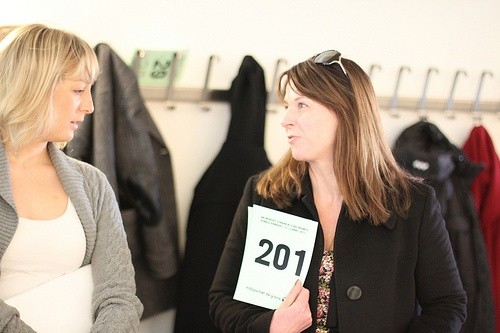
[209,51,467,333]
[0,23,145,333]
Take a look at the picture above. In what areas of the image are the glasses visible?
[313,49,349,79]
